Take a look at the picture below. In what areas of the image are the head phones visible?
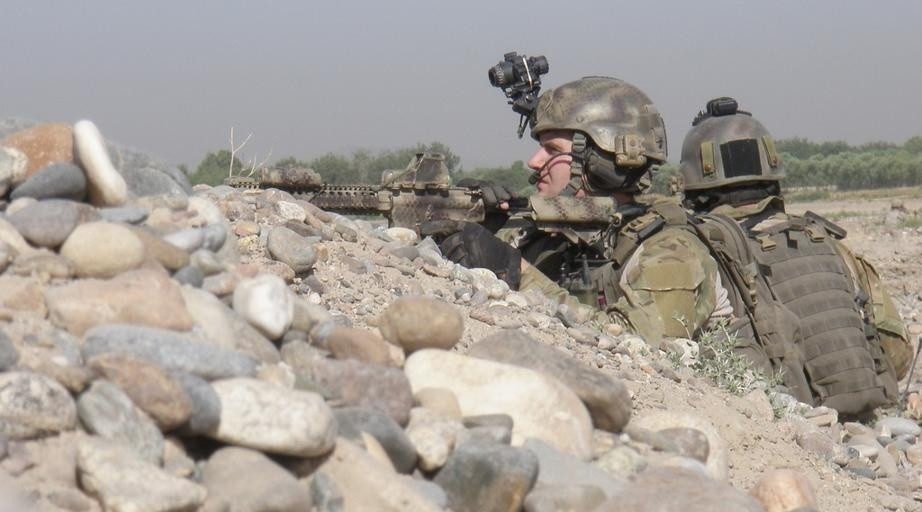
[585,145,628,191]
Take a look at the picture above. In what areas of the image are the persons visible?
[418,73,776,390]
[675,95,915,419]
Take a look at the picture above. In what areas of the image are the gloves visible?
[455,178,521,235]
[420,220,522,291]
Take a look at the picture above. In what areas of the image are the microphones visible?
[529,147,588,184]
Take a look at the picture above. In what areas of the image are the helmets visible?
[529,76,667,195]
[681,97,786,190]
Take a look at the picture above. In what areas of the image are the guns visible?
[223,152,618,265]
